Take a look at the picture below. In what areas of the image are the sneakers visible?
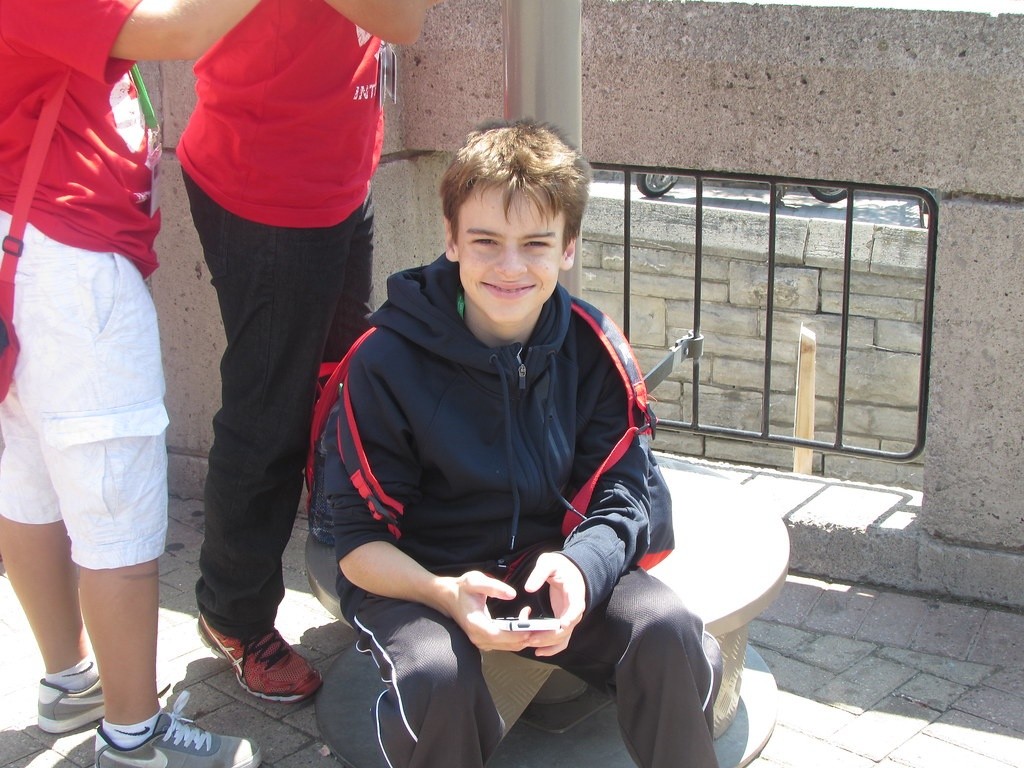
[94,714,261,767]
[37,654,172,734]
[197,612,323,704]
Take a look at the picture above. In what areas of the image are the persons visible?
[1,0,263,767]
[305,124,727,767]
[175,1,431,703]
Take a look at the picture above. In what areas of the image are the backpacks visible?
[563,297,677,576]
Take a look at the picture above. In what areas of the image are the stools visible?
[303,460,795,768]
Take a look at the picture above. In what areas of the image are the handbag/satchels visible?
[0,293,21,404]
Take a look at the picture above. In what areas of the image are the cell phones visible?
[497,613,558,633]
